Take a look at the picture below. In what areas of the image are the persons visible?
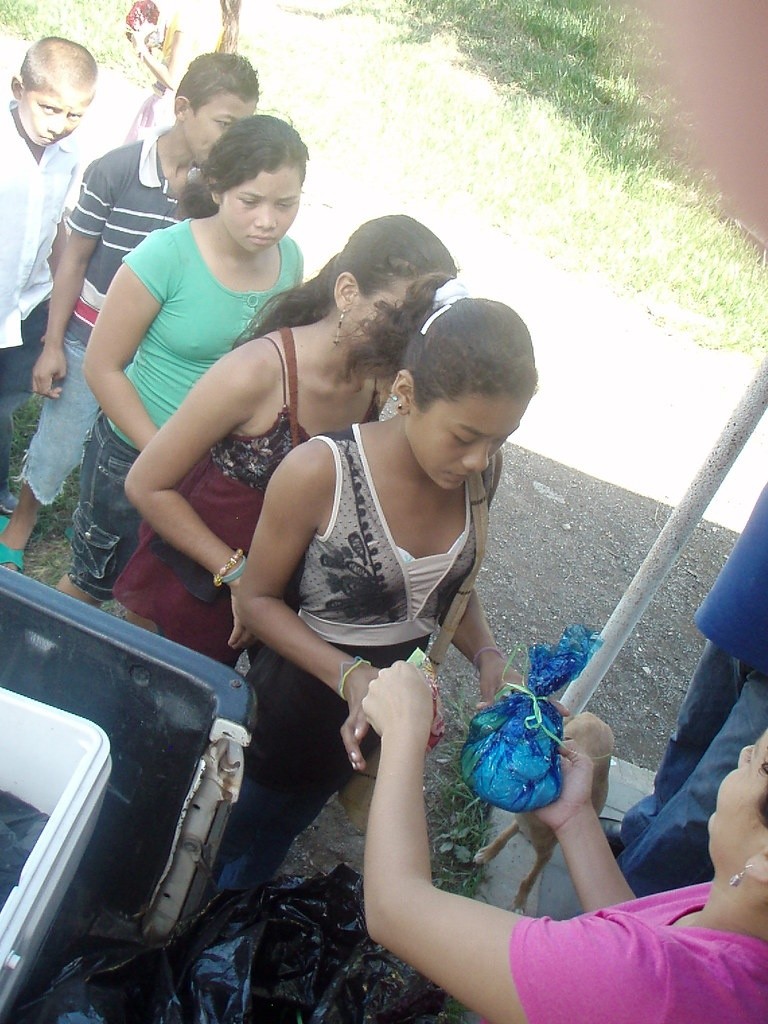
[55,115,308,632]
[111,209,455,678]
[124,1,194,144]
[0,51,259,571]
[604,480,768,899]
[359,661,768,1024]
[0,36,98,514]
[201,294,567,904]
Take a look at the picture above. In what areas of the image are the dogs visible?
[473,712,615,917]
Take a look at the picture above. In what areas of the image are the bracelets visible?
[337,656,371,699]
[137,51,143,58]
[214,548,247,587]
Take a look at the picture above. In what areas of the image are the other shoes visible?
[598,816,625,853]
[0,491,19,513]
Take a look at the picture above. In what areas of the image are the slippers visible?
[65,527,75,541]
[0,516,24,574]
